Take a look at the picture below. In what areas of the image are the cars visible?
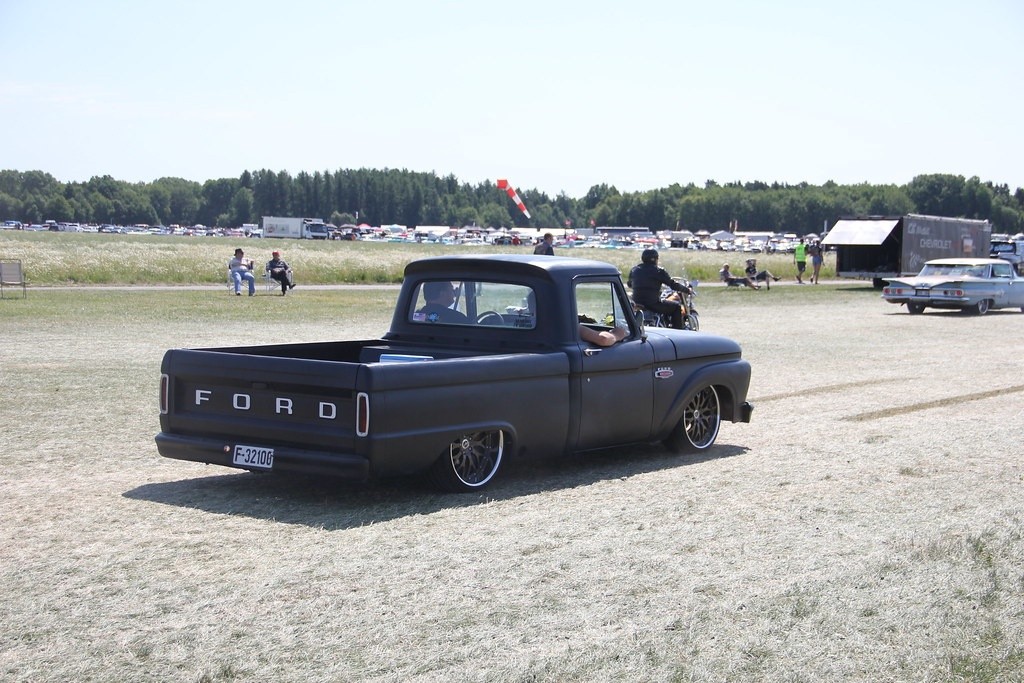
[326,224,820,254]
[881,257,1024,316]
[0,220,263,238]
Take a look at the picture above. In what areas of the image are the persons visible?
[746,259,781,290]
[528,291,626,346]
[794,239,810,284]
[267,251,296,296]
[724,264,761,290]
[230,249,255,296]
[504,236,519,244]
[809,241,826,284]
[534,233,554,255]
[416,232,421,243]
[29,221,32,226]
[627,249,692,330]
[420,281,467,324]
[455,234,481,239]
[806,244,815,247]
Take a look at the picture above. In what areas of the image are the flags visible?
[565,219,570,225]
[591,219,595,226]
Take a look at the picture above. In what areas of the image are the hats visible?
[272,251,279,254]
[234,249,243,255]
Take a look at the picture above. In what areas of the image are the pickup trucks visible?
[155,255,755,493]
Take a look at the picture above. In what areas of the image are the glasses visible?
[452,287,459,296]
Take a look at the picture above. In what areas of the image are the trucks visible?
[262,216,328,240]
[821,212,1024,289]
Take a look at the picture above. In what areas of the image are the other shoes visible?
[774,277,781,281]
[283,292,286,296]
[249,293,254,296]
[236,291,241,295]
[289,283,296,289]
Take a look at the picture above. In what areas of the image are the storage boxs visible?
[380,354,434,362]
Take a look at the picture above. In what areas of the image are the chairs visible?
[744,268,758,286]
[261,263,293,296]
[719,271,742,291]
[227,258,254,295]
[0,258,27,298]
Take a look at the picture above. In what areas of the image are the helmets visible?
[641,249,659,264]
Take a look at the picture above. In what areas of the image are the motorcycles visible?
[600,276,699,331]
